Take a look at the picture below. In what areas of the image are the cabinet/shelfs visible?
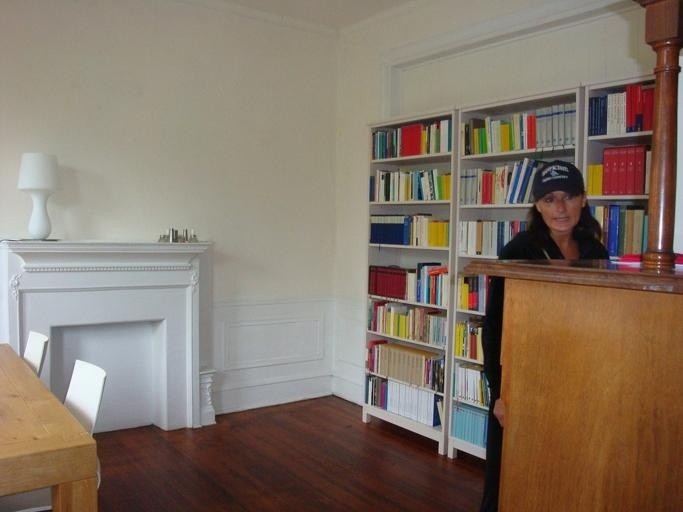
[360,69,660,462]
[442,266,503,461]
[459,85,581,261]
[363,109,457,456]
[584,75,656,263]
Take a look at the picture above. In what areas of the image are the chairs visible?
[0,330,107,512]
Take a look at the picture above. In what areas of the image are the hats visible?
[532,159,584,200]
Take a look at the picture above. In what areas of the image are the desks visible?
[0,341,99,511]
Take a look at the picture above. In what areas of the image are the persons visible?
[478,159,612,512]
